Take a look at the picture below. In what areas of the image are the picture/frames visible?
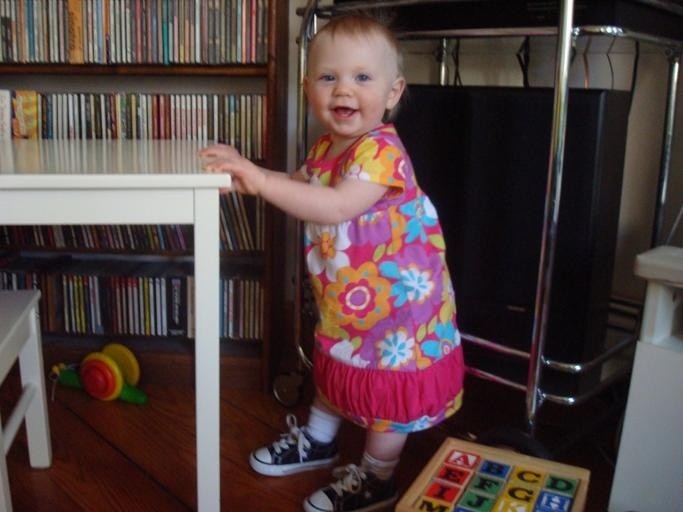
[395,436,593,512]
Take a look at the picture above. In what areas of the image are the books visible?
[3,0,269,162]
[1,188,271,337]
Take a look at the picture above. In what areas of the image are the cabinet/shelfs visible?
[1,0,276,359]
[293,0,682,440]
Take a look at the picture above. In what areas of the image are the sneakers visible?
[303,472,399,511]
[249,426,340,477]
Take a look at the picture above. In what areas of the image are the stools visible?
[0,288,53,512]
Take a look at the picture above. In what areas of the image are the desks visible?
[605,236,683,512]
[0,139,234,512]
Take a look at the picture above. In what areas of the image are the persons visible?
[197,16,469,511]
